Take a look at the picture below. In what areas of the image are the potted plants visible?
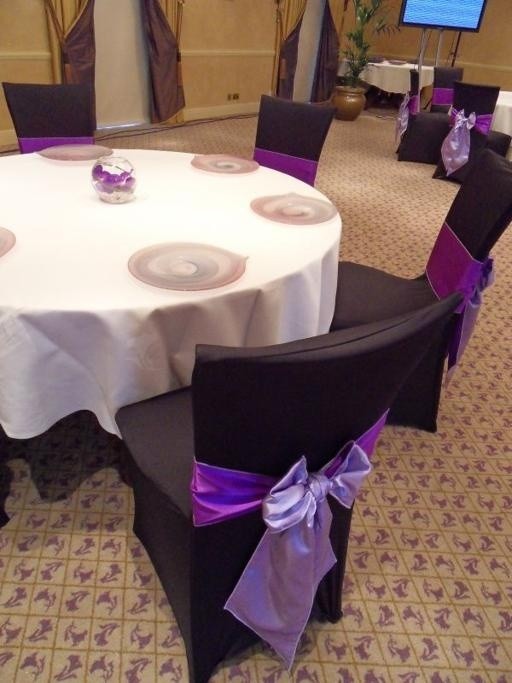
[332,0,402,122]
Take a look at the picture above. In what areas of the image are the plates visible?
[1,228,16,259]
[39,141,113,160]
[190,152,260,174]
[128,241,247,291]
[250,193,338,226]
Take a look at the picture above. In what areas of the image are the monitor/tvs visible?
[398,0,487,33]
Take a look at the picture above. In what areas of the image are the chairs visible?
[430,67,464,113]
[253,94,338,187]
[395,71,449,164]
[2,82,95,154]
[329,148,512,432]
[115,290,466,683]
[432,79,512,184]
